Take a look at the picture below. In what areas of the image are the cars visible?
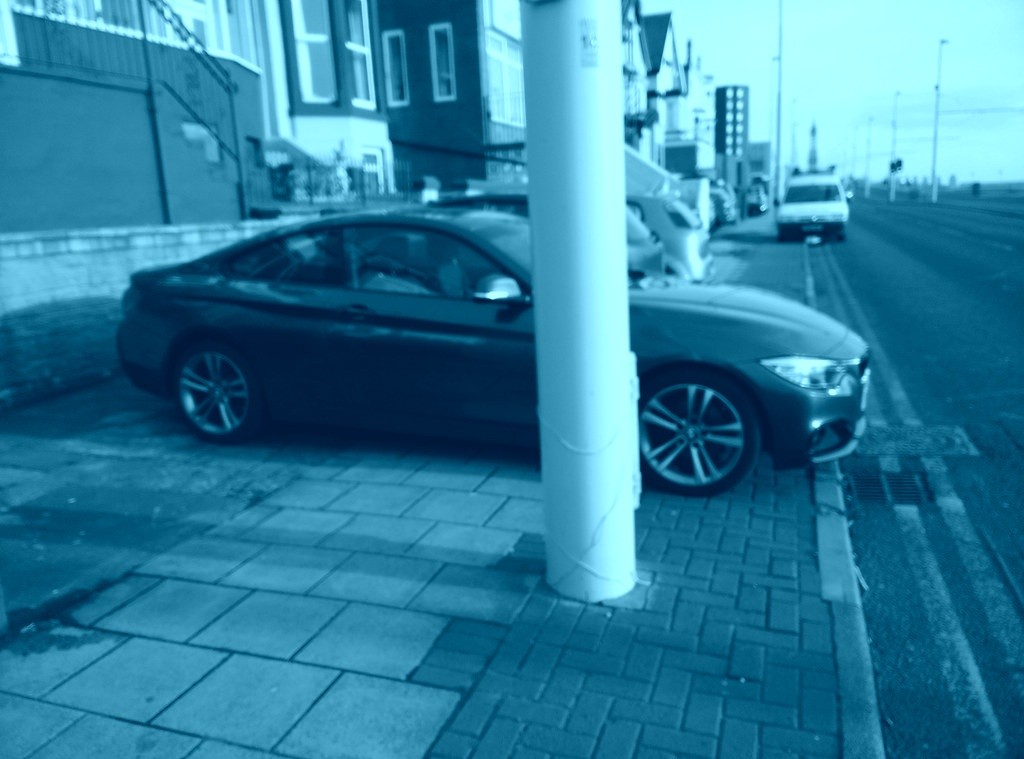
[115,201,872,498]
[358,168,768,291]
[775,171,855,242]
